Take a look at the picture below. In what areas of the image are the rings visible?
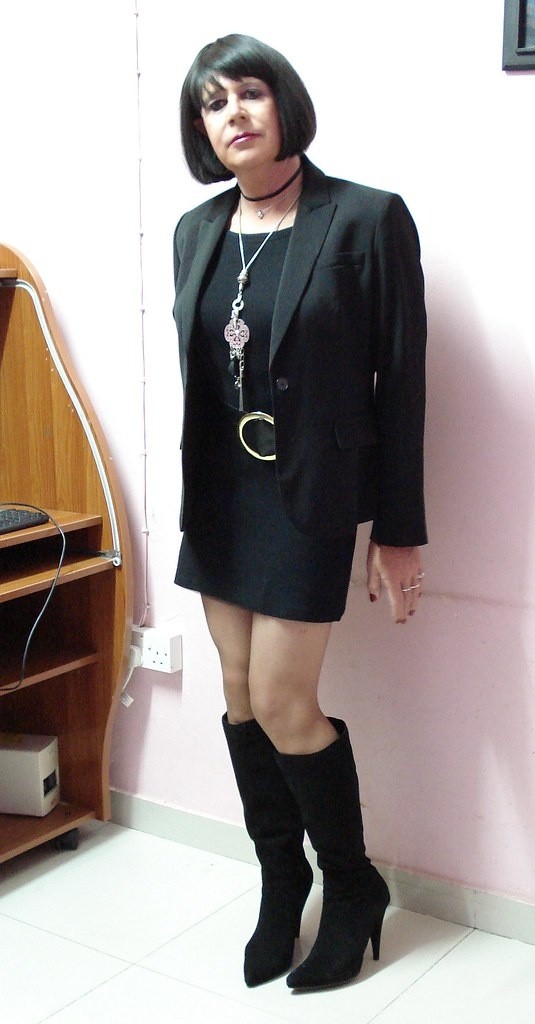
[402,583,420,592]
[416,573,425,579]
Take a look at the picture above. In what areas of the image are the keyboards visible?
[0,508,49,535]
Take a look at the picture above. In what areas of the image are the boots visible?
[274,717,391,990]
[221,711,314,987]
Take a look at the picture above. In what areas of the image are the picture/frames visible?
[502,0,535,70]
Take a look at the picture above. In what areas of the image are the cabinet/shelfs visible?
[0,243,135,865]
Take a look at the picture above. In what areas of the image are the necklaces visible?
[225,164,302,414]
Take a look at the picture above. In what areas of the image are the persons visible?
[171,33,427,993]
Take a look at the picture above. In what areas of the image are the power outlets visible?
[126,626,151,666]
